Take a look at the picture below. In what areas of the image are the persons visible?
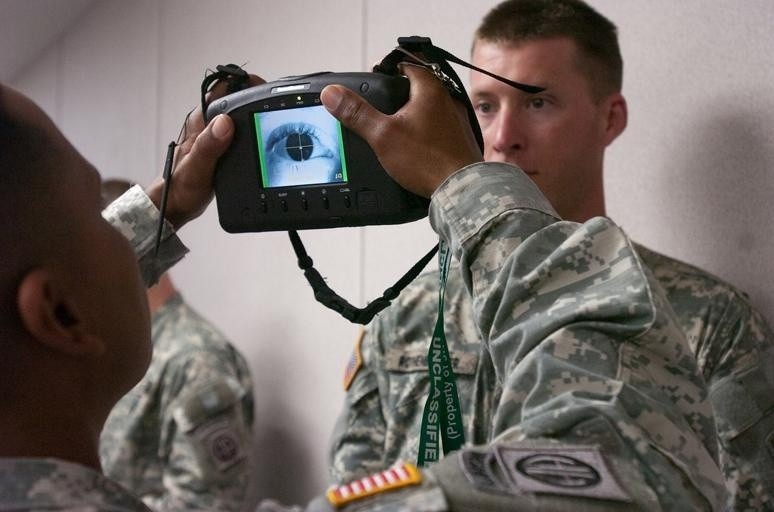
[95,177,255,511]
[0,63,730,511]
[324,0,773,511]
